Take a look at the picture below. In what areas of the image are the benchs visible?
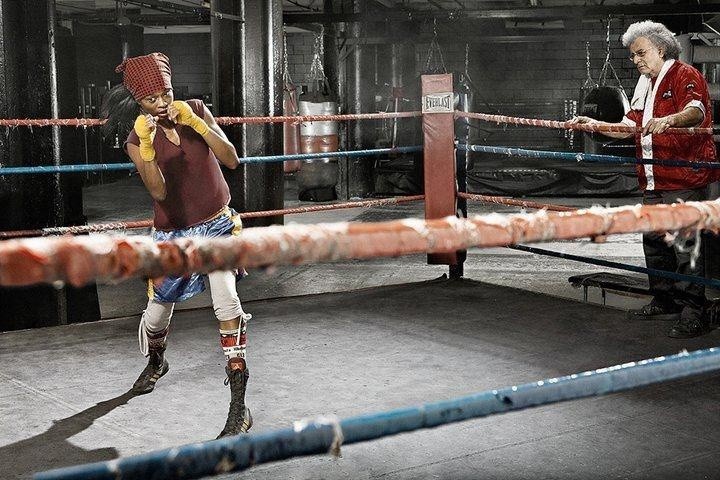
[566,272,720,330]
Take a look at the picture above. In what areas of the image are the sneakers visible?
[670,305,714,338]
[625,299,677,321]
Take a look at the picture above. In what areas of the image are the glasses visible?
[627,46,659,62]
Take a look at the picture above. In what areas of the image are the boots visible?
[213,366,252,441]
[132,344,170,394]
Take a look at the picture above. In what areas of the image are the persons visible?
[100,51,254,440]
[566,20,719,339]
